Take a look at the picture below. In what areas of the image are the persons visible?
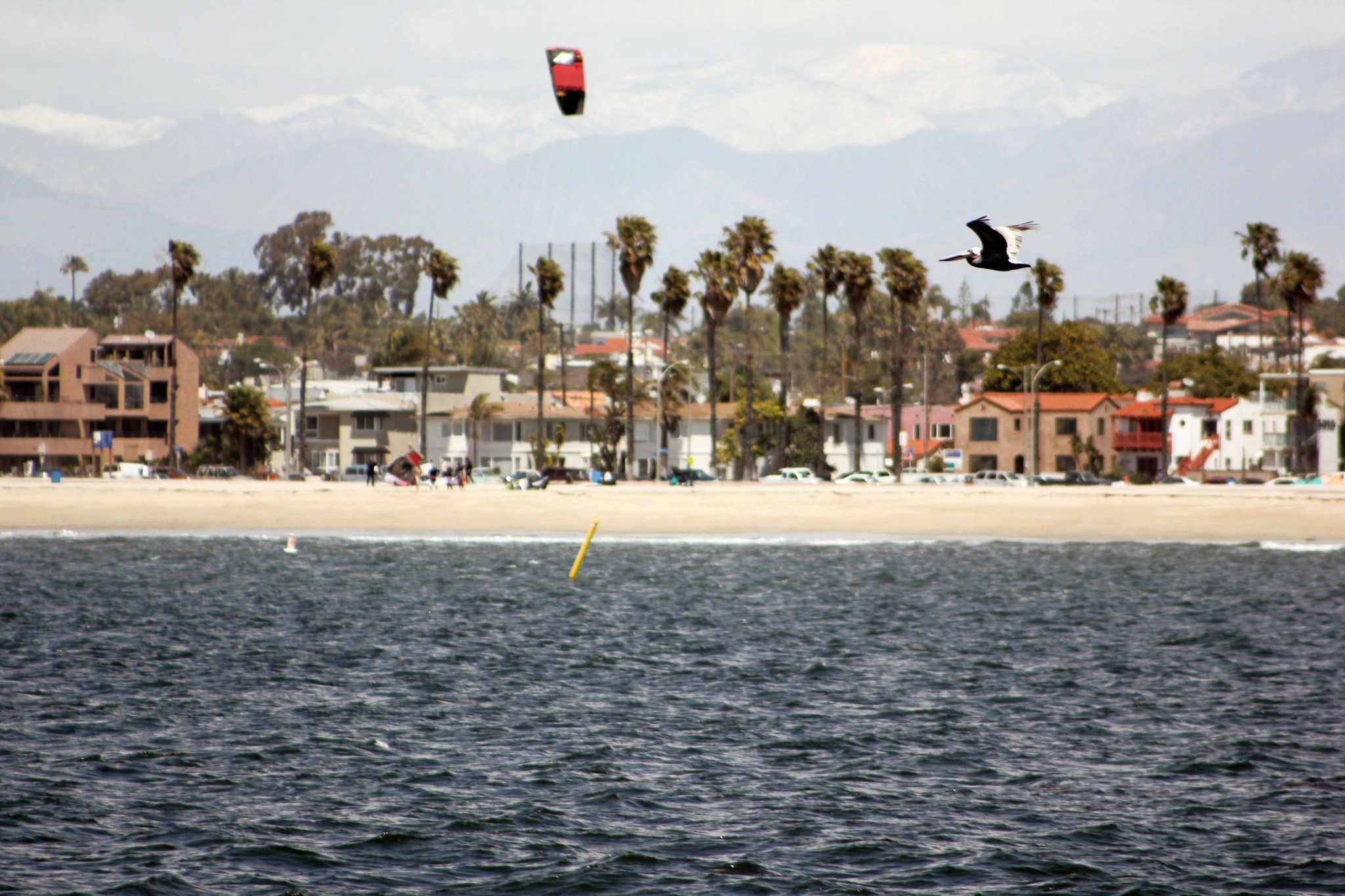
[428,456,474,489]
[365,455,377,486]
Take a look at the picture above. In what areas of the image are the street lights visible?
[655,360,688,484]
[1030,360,1064,486]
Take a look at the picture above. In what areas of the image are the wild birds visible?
[938,217,1038,271]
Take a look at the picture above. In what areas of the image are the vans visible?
[342,465,382,481]
[102,462,148,480]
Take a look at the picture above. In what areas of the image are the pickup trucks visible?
[1043,471,1111,485]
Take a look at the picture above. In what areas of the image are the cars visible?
[678,470,718,481]
[153,466,191,479]
[768,466,967,484]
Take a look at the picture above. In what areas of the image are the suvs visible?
[542,467,589,481]
[968,469,1027,487]
